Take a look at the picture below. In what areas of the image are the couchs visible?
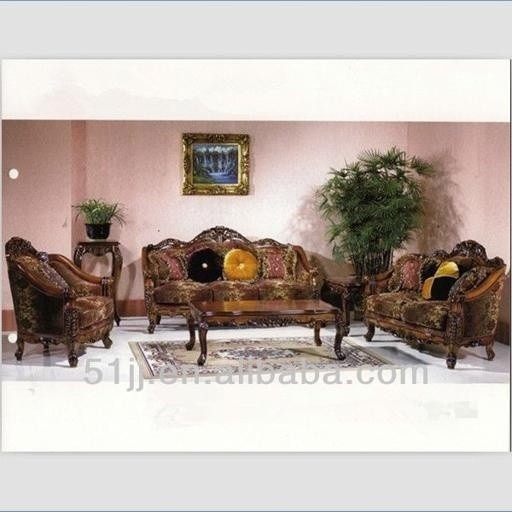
[358,237,507,370]
[138,224,328,334]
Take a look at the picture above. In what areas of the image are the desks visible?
[319,274,370,328]
[69,238,125,327]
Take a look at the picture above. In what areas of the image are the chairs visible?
[3,234,125,368]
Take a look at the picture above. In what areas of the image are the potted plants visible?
[70,195,129,239]
[311,140,443,323]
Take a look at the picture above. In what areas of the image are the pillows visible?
[221,248,260,282]
[387,251,497,306]
[34,259,78,300]
[183,247,222,283]
[149,250,188,285]
[255,245,297,283]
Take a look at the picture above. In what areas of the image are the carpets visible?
[124,333,402,382]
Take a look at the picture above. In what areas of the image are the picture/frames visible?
[180,130,251,196]
